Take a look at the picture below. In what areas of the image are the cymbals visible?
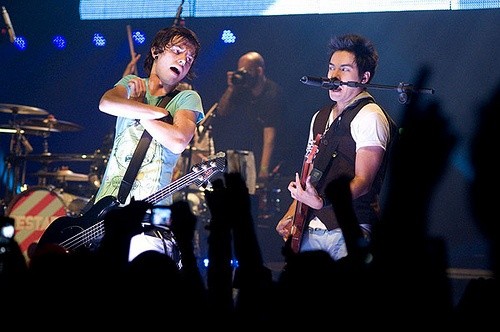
[0,103,48,115]
[7,119,86,132]
[32,169,89,181]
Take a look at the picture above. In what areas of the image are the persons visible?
[94,25,205,263]
[275,32,392,261]
[216,51,291,180]
[0,56,499,332]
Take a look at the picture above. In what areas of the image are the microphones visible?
[2,7,16,42]
[300,75,339,90]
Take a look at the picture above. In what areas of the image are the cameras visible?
[152,206,170,225]
[231,68,253,87]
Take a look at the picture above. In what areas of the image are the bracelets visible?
[259,166,268,172]
[135,118,140,124]
[154,111,174,126]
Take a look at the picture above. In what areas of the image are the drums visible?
[89,136,114,190]
[4,185,91,263]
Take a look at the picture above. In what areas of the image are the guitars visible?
[37,151,229,253]
[291,133,322,254]
